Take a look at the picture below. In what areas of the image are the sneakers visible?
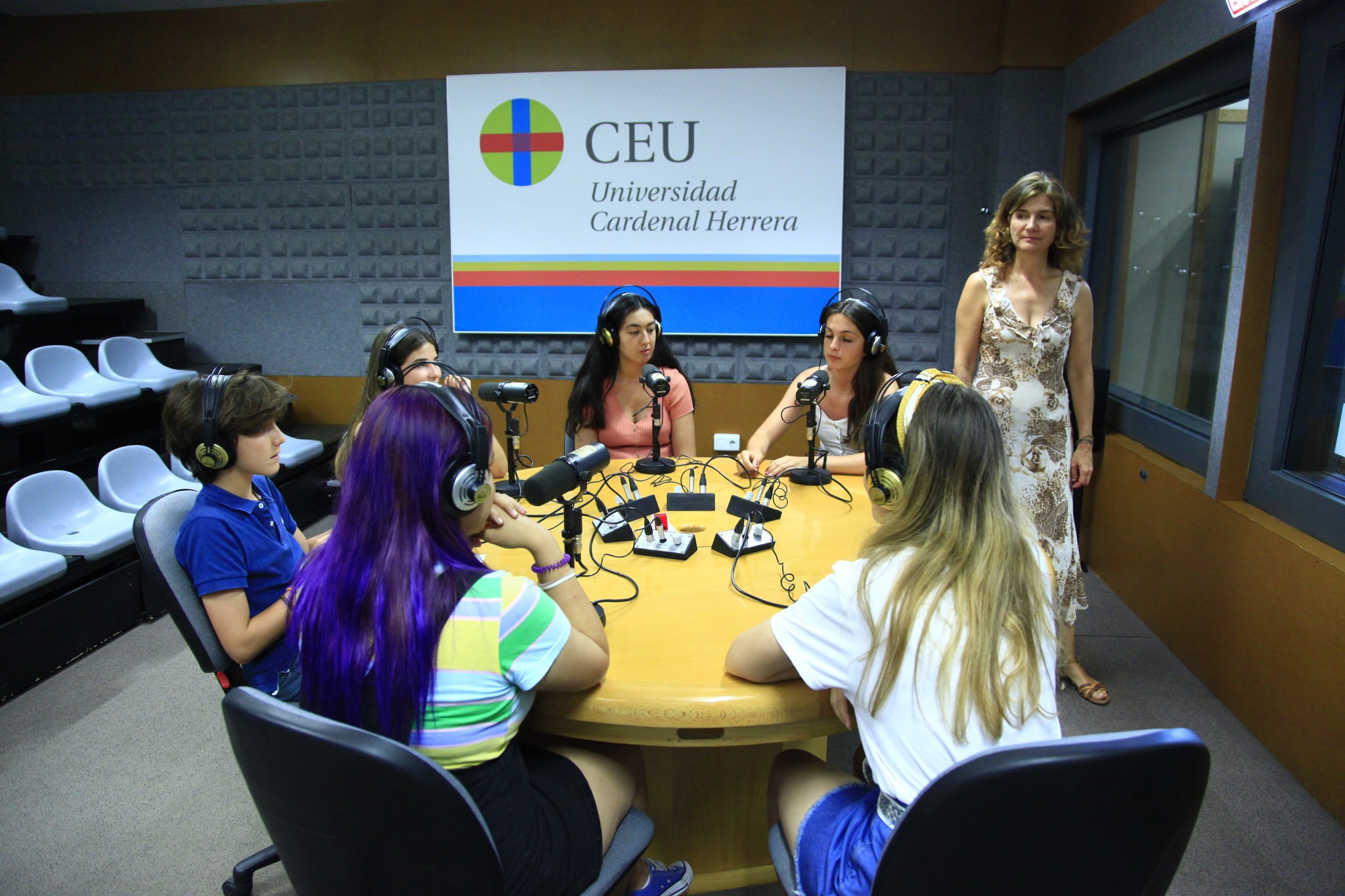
[624,857,693,896]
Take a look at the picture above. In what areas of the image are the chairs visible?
[221,685,655,896]
[135,488,286,896]
[767,728,1212,896]
[0,263,324,708]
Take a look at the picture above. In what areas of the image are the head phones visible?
[597,284,662,347]
[862,369,958,506]
[194,364,237,470]
[386,360,491,517]
[375,317,436,391]
[817,286,889,356]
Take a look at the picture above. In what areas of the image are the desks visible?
[468,456,882,895]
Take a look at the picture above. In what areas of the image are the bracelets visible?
[537,568,577,590]
[1076,435,1095,445]
[279,593,296,614]
[531,553,572,574]
[284,585,299,596]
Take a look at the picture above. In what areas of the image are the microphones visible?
[796,370,829,406]
[523,443,611,505]
[641,363,670,397]
[477,382,539,405]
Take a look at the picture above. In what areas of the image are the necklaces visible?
[831,371,856,394]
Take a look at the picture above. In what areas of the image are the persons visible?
[565,295,696,459]
[287,384,693,896]
[161,372,332,702]
[736,300,902,478]
[352,322,508,479]
[953,172,1110,704]
[725,382,1062,896]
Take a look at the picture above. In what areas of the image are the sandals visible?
[1055,656,1110,705]
